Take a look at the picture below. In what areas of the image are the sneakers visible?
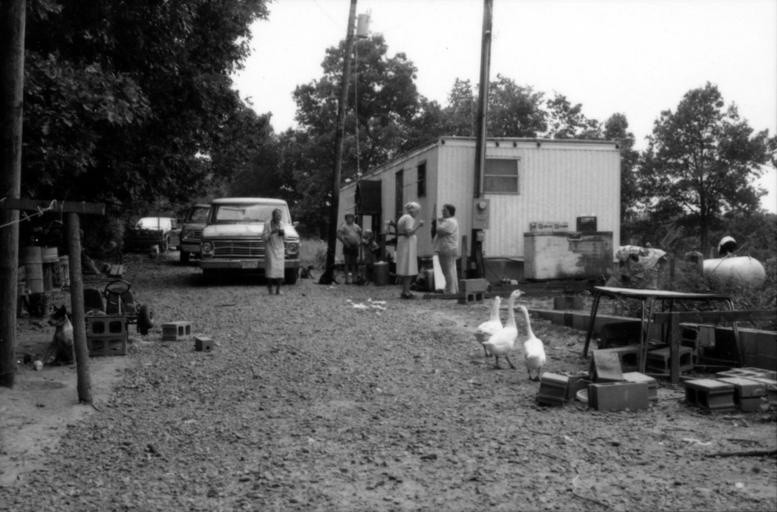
[441,294,460,299]
[401,293,417,299]
[345,279,357,284]
[269,290,284,295]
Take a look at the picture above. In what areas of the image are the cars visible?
[134,196,302,285]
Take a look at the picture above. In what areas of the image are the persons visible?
[432,202,460,294]
[365,228,381,278]
[337,213,363,285]
[396,201,425,297]
[260,208,289,294]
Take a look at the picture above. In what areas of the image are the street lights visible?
[319,13,372,285]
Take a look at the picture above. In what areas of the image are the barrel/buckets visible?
[60,256,70,287]
[373,261,389,285]
[44,261,60,289]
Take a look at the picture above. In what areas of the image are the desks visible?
[584,285,745,384]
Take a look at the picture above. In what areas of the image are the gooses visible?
[513,306,546,382]
[474,296,505,359]
[482,289,526,370]
